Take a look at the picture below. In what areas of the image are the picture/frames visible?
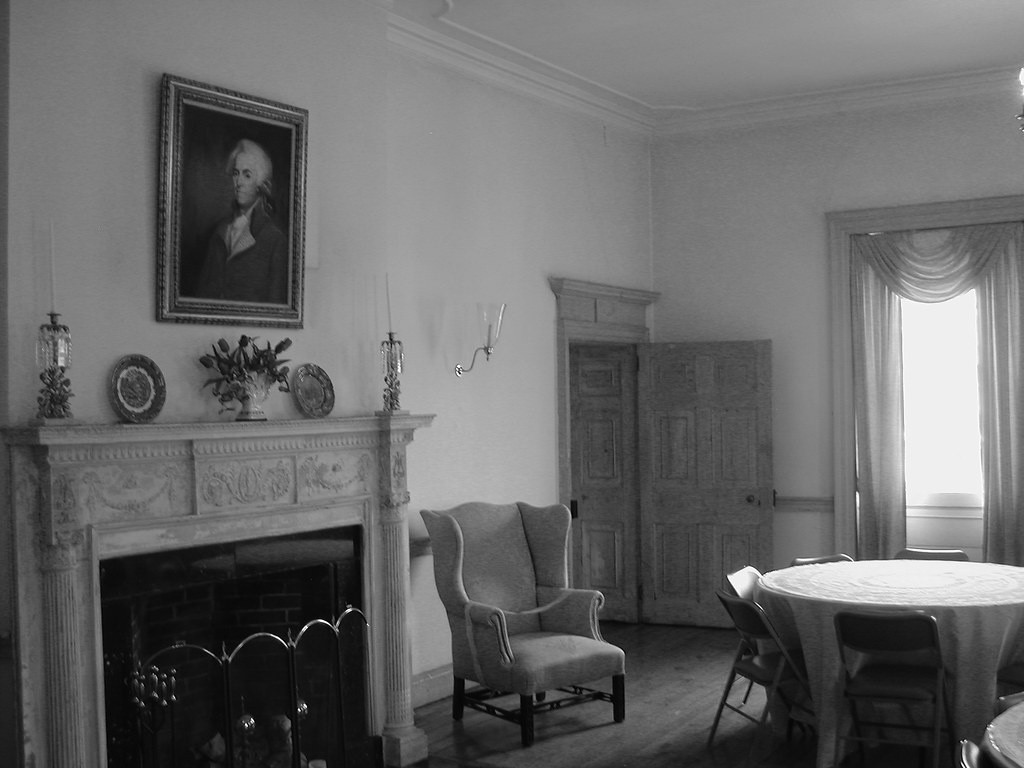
[155,72,309,330]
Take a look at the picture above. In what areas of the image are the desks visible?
[977,702,1024,768]
[751,559,1024,768]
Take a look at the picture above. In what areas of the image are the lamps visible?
[455,301,507,378]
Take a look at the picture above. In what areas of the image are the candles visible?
[48,212,58,313]
[386,272,392,332]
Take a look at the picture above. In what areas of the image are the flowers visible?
[198,334,293,415]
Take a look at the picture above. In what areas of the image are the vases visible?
[231,372,271,421]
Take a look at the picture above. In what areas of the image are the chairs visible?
[993,691,1024,717]
[894,548,969,561]
[725,565,763,703]
[791,552,854,566]
[958,738,980,768]
[833,611,957,768]
[706,588,814,768]
[419,501,626,749]
